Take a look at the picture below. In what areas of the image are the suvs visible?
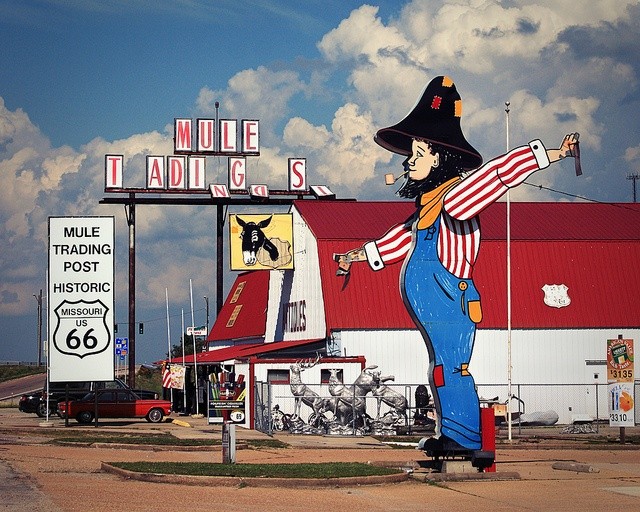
[43,376,158,415]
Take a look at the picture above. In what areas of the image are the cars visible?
[58,390,173,423]
[19,392,56,416]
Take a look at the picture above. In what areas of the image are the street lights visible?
[33,293,42,366]
[203,296,208,354]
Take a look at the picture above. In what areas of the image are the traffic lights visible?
[115,324,118,332]
[139,323,143,333]
